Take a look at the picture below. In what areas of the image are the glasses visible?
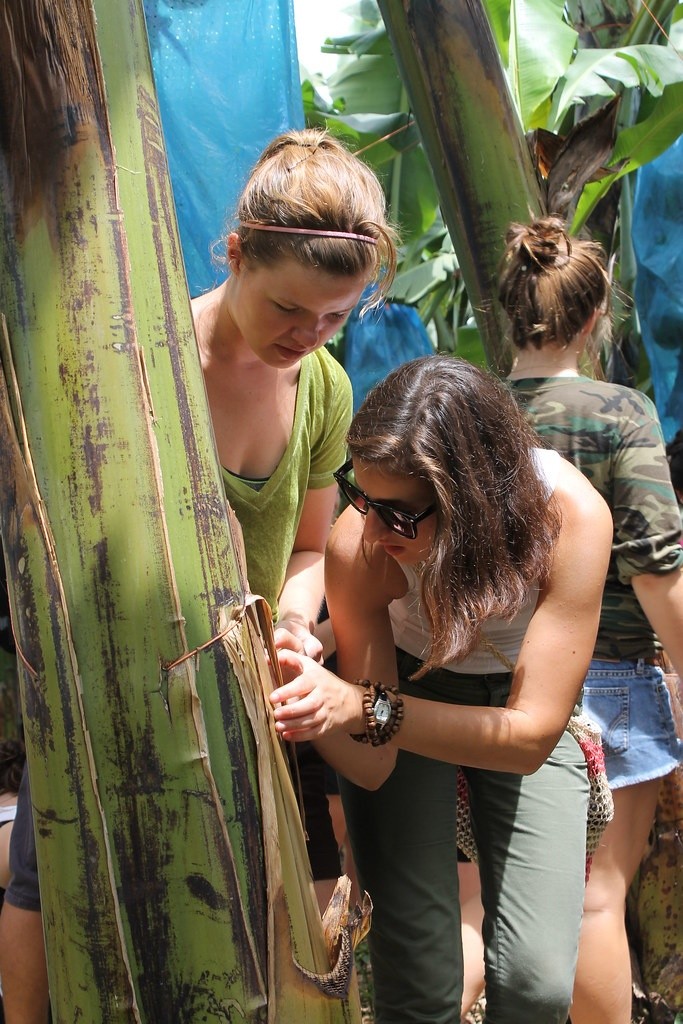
[332,460,436,541]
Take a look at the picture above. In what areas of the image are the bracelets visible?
[352,678,404,749]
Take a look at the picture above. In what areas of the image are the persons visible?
[498,216,682,1024]
[266,355,615,1023]
[0,129,400,1024]
[0,596,487,1022]
